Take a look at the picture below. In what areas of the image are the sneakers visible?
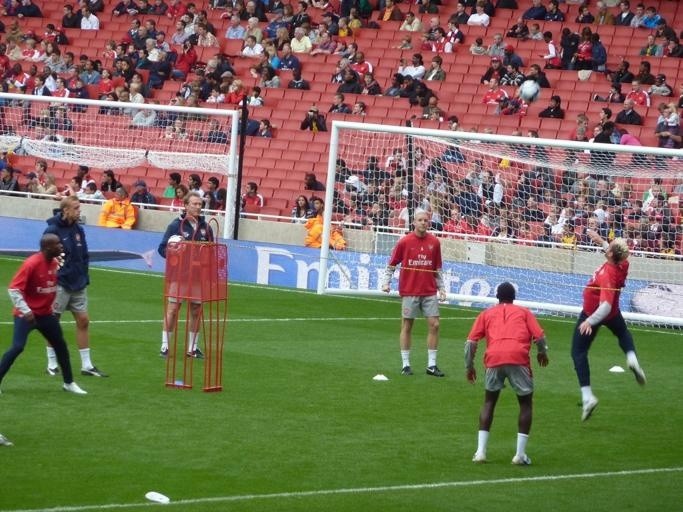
[580,397,598,425]
[628,360,647,386]
[511,454,532,465]
[45,367,62,375]
[400,365,414,376]
[185,347,205,359]
[80,367,109,378]
[63,382,88,395]
[159,349,169,358]
[426,365,445,377]
[472,451,487,464]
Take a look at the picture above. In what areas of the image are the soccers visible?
[519,79,541,102]
[167,235,187,251]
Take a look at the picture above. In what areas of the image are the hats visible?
[53,27,64,33]
[23,171,37,179]
[221,71,233,78]
[132,180,147,188]
[345,175,361,184]
[655,18,667,24]
[501,45,515,54]
[309,106,319,112]
[490,55,503,62]
[320,11,334,18]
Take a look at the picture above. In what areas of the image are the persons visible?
[155,192,212,360]
[379,208,447,378]
[0,233,88,396]
[570,228,647,423]
[461,281,550,464]
[1,0,683,259]
[40,194,108,377]
